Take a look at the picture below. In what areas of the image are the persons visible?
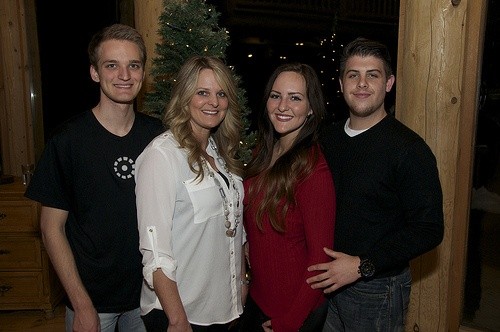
[133,55,250,332]
[317,38,444,332]
[240,63,335,332]
[24,24,165,332]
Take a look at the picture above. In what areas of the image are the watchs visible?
[357,254,375,281]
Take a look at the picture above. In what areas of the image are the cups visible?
[21,161,35,185]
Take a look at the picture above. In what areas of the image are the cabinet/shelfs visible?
[0,192,64,319]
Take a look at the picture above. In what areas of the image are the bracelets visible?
[240,273,251,287]
